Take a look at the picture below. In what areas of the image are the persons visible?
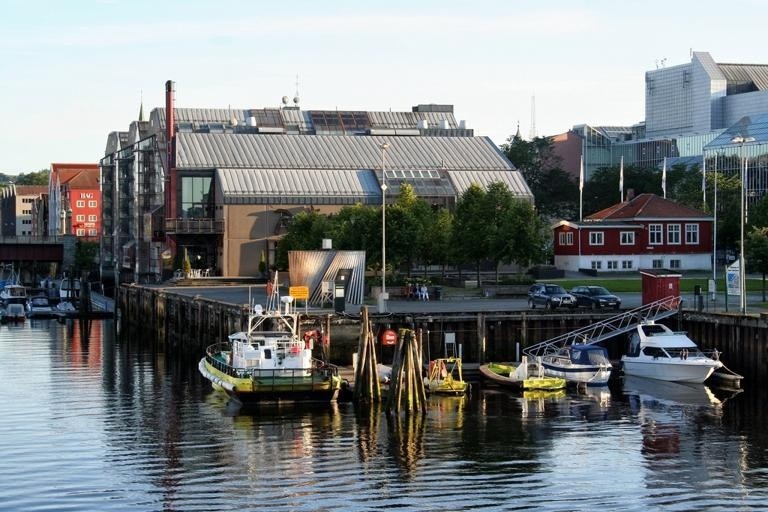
[421,284,429,300]
[405,283,415,300]
[712,348,718,360]
[413,283,421,300]
[680,349,686,360]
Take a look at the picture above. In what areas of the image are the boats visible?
[535,344,614,385]
[195,267,473,403]
[0,261,82,321]
[618,320,722,385]
[477,354,567,391]
[712,371,745,389]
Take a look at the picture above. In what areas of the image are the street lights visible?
[377,140,390,311]
[732,135,756,314]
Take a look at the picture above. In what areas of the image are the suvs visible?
[528,283,577,310]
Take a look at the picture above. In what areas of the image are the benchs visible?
[448,295,464,300]
[386,286,442,300]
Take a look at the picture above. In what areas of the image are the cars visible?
[570,285,622,312]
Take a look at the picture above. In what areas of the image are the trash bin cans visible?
[695,285,701,295]
[434,287,442,300]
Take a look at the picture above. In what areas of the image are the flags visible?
[660,157,666,192]
[579,156,586,190]
[619,157,624,192]
